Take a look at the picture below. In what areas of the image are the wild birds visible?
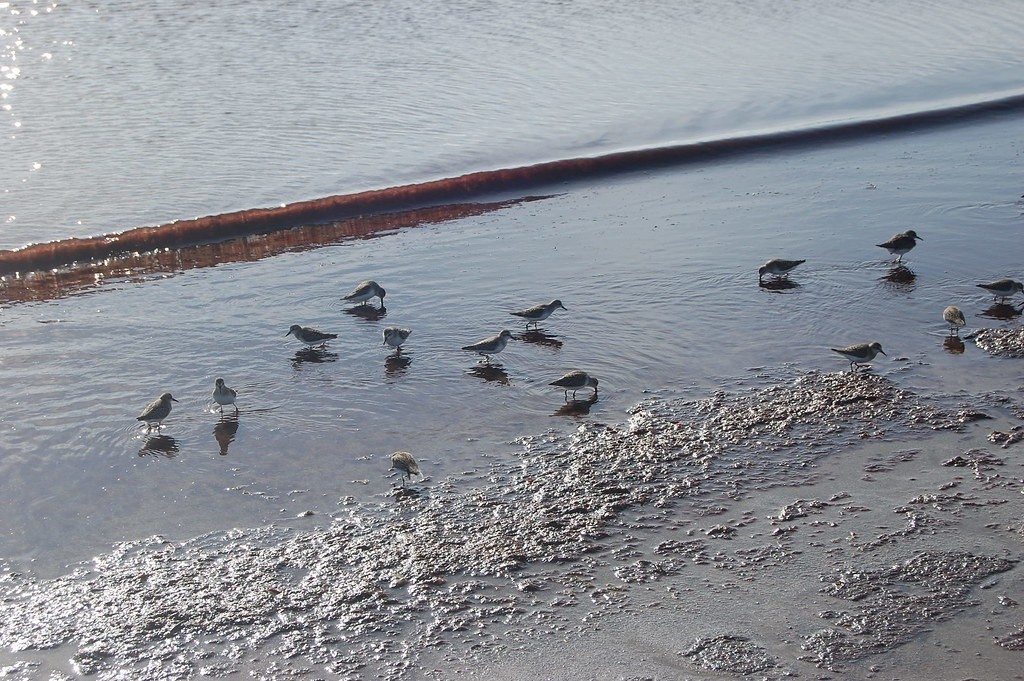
[460,329,519,359]
[758,259,809,283]
[975,279,1024,306]
[830,341,886,375]
[135,392,179,436]
[548,370,599,400]
[381,326,414,357]
[942,305,967,334]
[509,299,568,331]
[211,376,241,415]
[387,451,424,485]
[340,280,386,308]
[283,323,340,350]
[875,228,925,265]
